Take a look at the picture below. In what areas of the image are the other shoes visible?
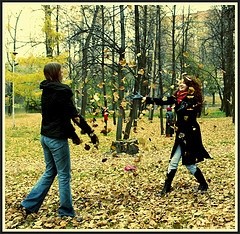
[20,204,34,219]
[60,216,83,222]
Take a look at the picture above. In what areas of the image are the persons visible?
[129,74,214,197]
[166,107,176,137]
[102,106,108,126]
[120,100,127,123]
[16,65,101,217]
[5,94,13,115]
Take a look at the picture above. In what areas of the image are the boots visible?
[160,169,177,195]
[191,167,208,191]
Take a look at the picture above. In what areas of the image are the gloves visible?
[131,91,143,100]
